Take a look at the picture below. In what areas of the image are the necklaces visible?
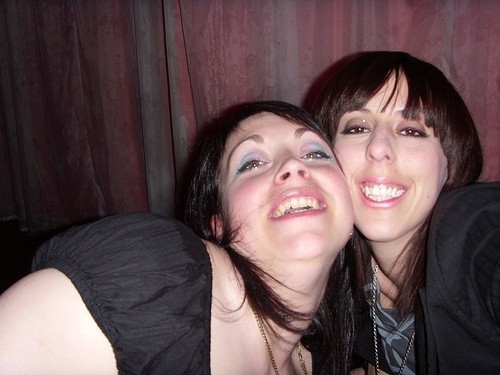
[250,296,311,375]
[370,256,417,375]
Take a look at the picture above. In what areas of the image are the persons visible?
[300,50,499,375]
[0,100,377,375]
